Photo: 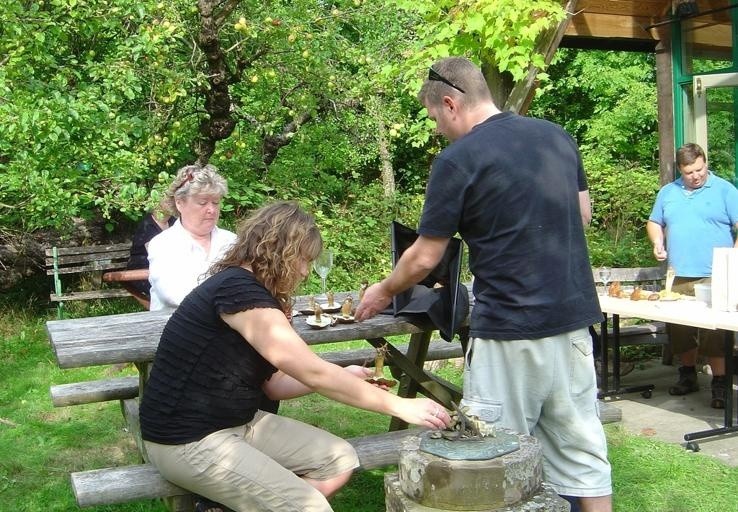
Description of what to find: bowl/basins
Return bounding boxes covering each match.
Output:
[693,283,712,305]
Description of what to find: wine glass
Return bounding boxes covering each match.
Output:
[314,249,332,293]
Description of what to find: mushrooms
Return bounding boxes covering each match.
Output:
[292,280,370,330]
[609,266,681,301]
[365,343,398,387]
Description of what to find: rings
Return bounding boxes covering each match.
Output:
[434,408,440,417]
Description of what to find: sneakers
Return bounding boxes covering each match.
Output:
[712,384,727,408]
[669,377,699,395]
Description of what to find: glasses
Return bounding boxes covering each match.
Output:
[428,66,465,93]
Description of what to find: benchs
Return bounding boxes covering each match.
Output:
[51,340,462,408]
[43,242,142,319]
[69,428,428,508]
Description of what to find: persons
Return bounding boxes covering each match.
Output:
[352,57,613,511]
[140,197,453,511]
[125,190,177,300]
[646,142,738,410]
[145,163,241,309]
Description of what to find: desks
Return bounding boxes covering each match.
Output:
[595,287,738,455]
[45,280,479,512]
